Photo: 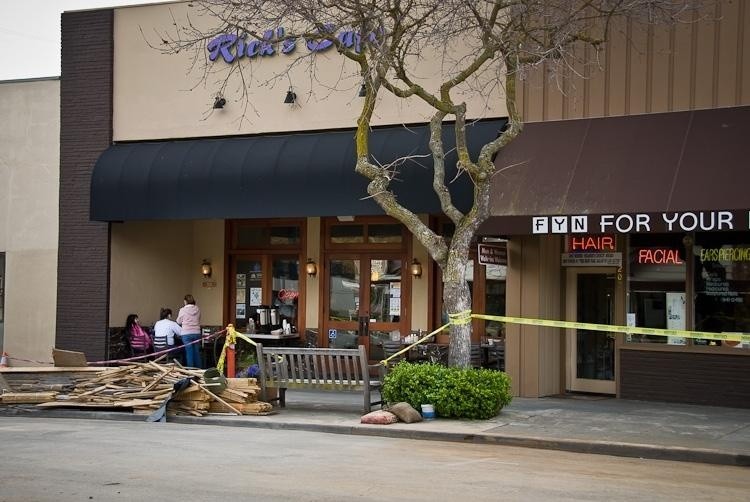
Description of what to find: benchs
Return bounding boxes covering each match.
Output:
[382,342,504,371]
[255,343,388,416]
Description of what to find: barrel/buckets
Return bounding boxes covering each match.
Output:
[421,404,435,420]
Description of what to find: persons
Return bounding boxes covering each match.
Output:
[154,308,181,354]
[125,314,152,350]
[176,294,202,368]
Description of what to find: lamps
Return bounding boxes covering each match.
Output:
[201,259,212,278]
[306,257,316,277]
[358,83,367,97]
[214,96,225,108]
[411,257,422,279]
[283,91,296,102]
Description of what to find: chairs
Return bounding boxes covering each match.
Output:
[127,328,220,368]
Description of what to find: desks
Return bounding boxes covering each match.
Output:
[239,332,299,346]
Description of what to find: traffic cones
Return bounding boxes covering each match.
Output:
[0,352,9,369]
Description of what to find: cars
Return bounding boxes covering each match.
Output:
[330,315,383,358]
